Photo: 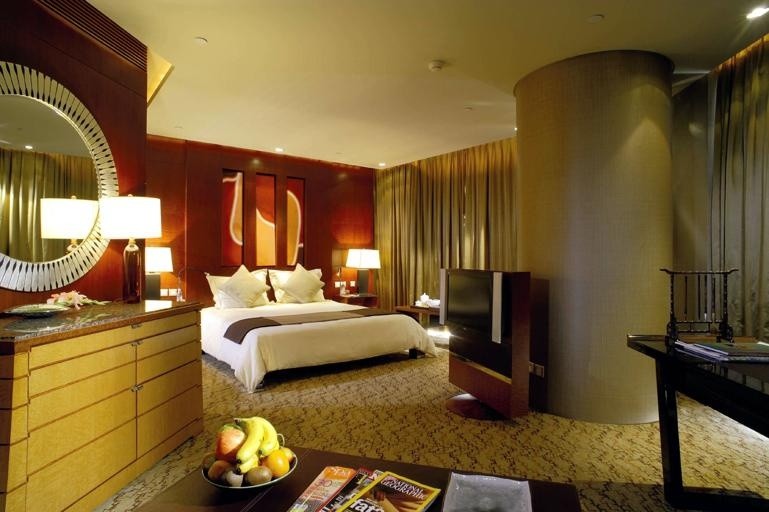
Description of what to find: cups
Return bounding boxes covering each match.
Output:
[415,290,440,307]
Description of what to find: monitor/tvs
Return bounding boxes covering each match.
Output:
[440,268,506,345]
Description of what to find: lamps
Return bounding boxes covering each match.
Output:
[346,249,381,296]
[145,247,173,300]
[102,194,163,303]
[40,196,98,255]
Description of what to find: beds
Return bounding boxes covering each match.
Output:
[198,299,438,393]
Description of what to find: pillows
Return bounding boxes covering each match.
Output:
[204,263,326,309]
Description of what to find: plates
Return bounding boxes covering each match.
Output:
[200,444,297,489]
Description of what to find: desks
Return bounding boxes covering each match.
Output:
[394,306,440,326]
[135,446,582,512]
[627,333,769,512]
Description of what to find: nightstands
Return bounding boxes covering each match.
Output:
[332,295,381,308]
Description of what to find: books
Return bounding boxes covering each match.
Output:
[674,338,769,363]
[283,465,442,512]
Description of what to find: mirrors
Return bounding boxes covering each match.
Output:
[1,62,119,292]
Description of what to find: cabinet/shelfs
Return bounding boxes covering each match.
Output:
[445,271,550,421]
[0,301,216,511]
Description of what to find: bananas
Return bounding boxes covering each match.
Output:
[233,415,279,475]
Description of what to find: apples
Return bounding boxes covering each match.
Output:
[215,425,249,463]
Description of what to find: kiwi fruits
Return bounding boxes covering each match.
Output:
[242,466,273,485]
[220,467,244,487]
